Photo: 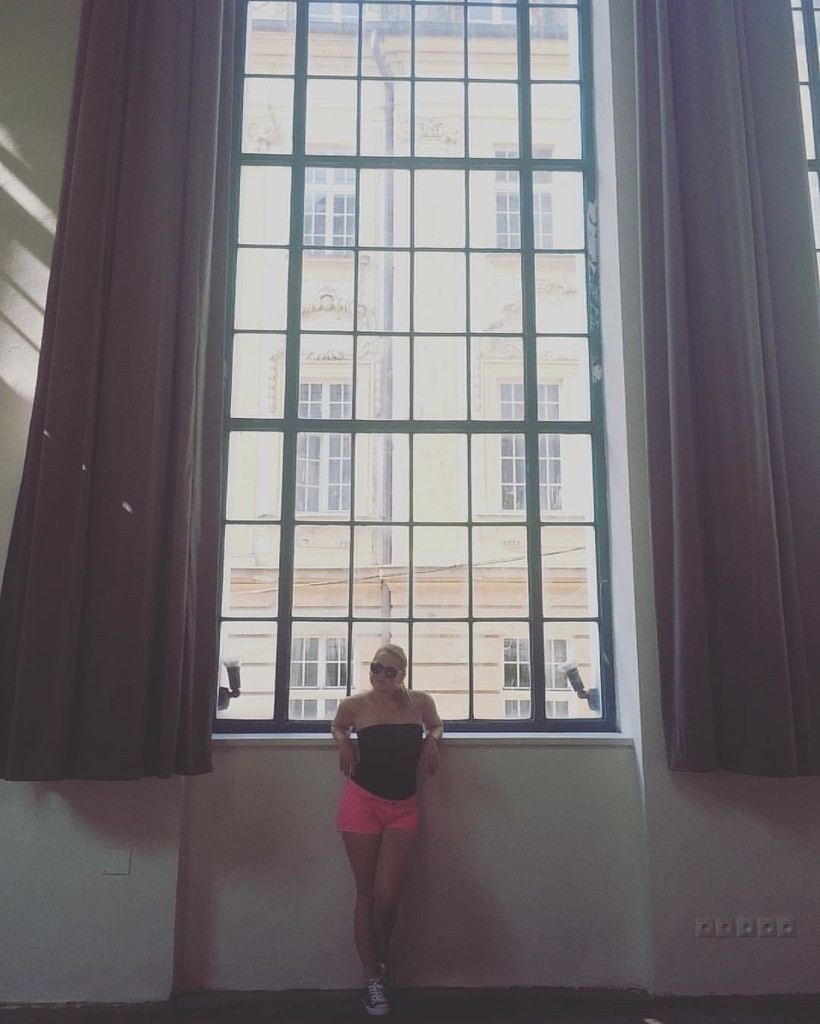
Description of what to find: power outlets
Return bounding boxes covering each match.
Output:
[755,917,777,937]
[715,918,735,938]
[776,916,797,937]
[735,917,756,937]
[695,918,714,936]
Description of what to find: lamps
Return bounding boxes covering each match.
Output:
[217,658,243,711]
[559,659,600,710]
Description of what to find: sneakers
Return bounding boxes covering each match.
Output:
[362,983,391,1016]
[378,962,390,983]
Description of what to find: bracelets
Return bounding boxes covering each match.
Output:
[425,733,439,743]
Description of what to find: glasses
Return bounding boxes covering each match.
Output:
[371,662,402,678]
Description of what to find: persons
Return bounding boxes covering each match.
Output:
[331,644,444,1015]
